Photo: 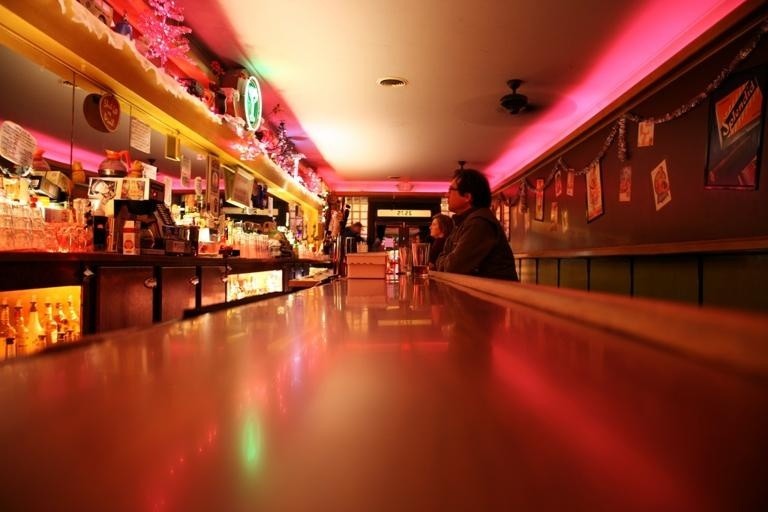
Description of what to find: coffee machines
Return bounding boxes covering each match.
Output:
[113,199,166,255]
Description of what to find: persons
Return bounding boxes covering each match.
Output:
[425,215,454,272]
[345,221,364,244]
[412,233,427,243]
[434,170,520,282]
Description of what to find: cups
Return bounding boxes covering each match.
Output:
[409,276,432,313]
[411,242,431,276]
[0,197,109,250]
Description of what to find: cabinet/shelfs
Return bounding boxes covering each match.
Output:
[0,0,336,361]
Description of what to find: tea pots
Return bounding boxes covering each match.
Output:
[99,151,131,173]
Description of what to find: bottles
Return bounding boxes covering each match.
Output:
[341,255,348,276]
[0,299,82,361]
[177,194,270,255]
